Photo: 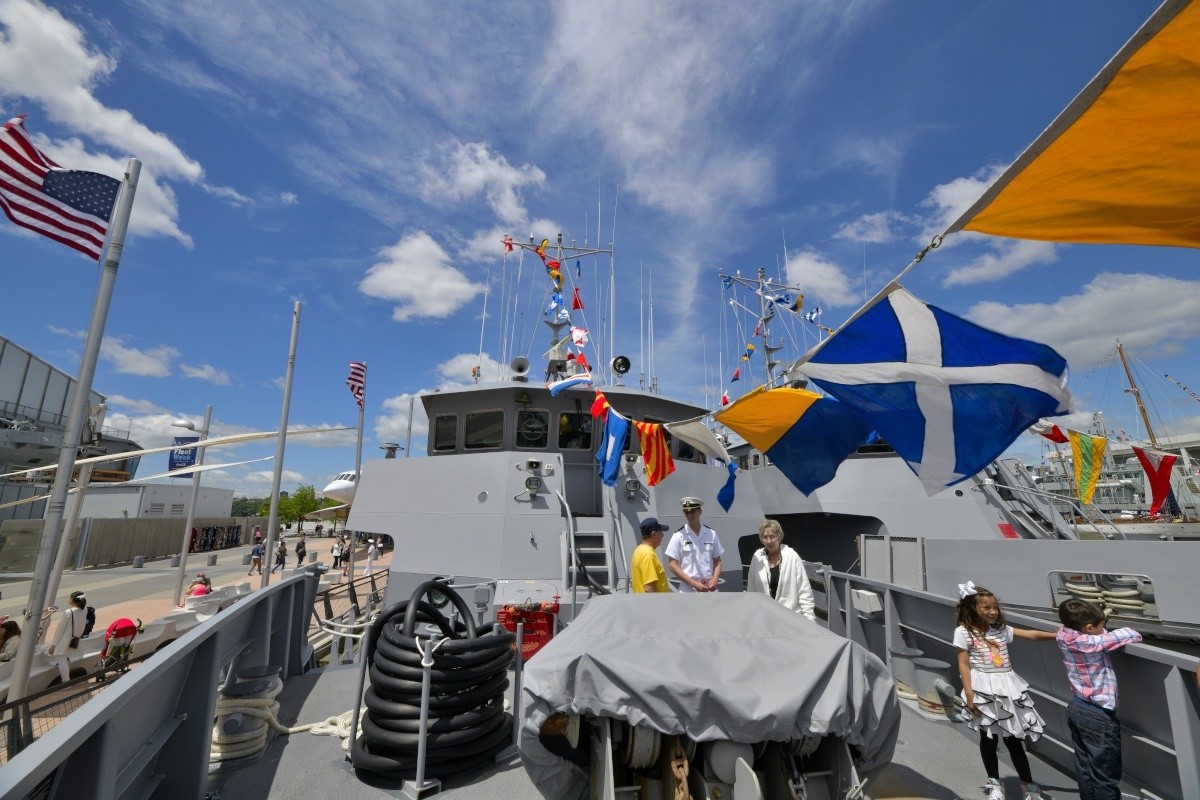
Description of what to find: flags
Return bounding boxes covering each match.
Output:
[1028,420,1069,443]
[710,381,877,497]
[345,361,367,409]
[718,273,834,406]
[1066,429,1108,505]
[961,0,1200,246]
[662,420,741,513]
[0,113,122,260]
[500,238,629,487]
[793,279,1075,497]
[631,420,676,486]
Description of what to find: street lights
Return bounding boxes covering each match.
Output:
[170,404,214,607]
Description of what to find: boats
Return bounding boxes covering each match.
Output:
[1027,337,1200,542]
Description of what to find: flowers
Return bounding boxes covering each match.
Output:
[958,580,976,599]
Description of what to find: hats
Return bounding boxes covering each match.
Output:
[679,497,704,512]
[192,577,203,582]
[368,538,375,544]
[639,518,669,532]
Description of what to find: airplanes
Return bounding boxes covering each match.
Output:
[303,469,362,520]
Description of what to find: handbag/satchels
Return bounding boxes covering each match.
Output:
[69,636,80,648]
[298,548,304,555]
[378,543,383,548]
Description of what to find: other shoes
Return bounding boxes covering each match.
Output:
[258,573,261,574]
[176,605,184,609]
[982,777,1006,800]
[1020,784,1043,800]
[248,573,253,576]
[60,682,71,691]
[55,657,70,665]
[342,574,347,577]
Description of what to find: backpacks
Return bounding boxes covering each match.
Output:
[330,546,334,553]
[80,606,95,638]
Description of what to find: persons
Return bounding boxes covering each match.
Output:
[0,591,9,623]
[295,536,306,568]
[375,535,384,557]
[665,500,724,593]
[953,585,1057,800]
[254,529,263,544]
[247,539,267,576]
[271,542,288,574]
[332,537,351,576]
[747,519,817,623]
[340,530,361,548]
[299,528,305,540]
[314,525,334,538]
[48,591,88,692]
[274,527,287,564]
[176,572,212,608]
[1056,598,1142,800]
[359,540,375,576]
[630,518,668,594]
